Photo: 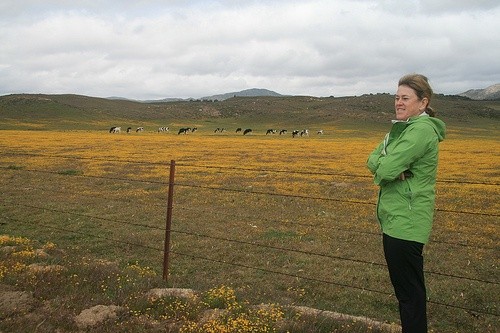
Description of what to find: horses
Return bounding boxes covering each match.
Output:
[178,128,198,135]
[214,127,225,134]
[109,126,144,134]
[157,126,169,134]
[235,127,252,135]
[265,128,324,138]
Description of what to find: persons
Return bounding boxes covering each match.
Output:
[365,74,446,332]
